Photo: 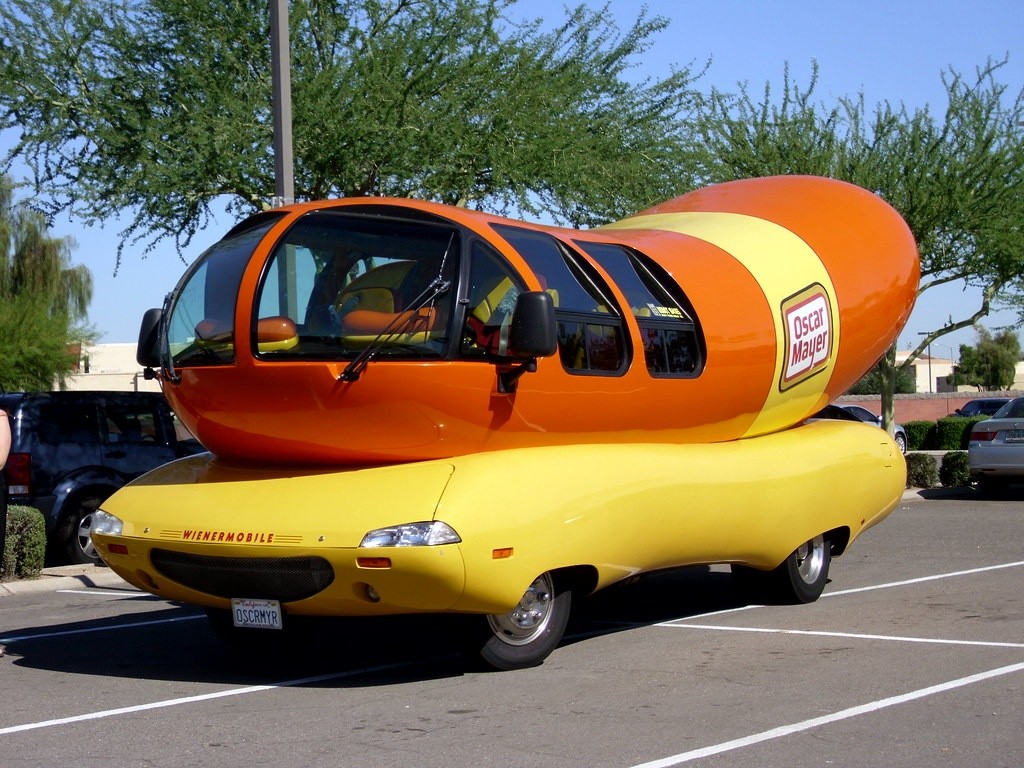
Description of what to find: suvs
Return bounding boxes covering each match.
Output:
[1,390,210,565]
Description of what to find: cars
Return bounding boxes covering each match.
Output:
[955,398,1012,418]
[968,396,1024,493]
[833,403,908,456]
